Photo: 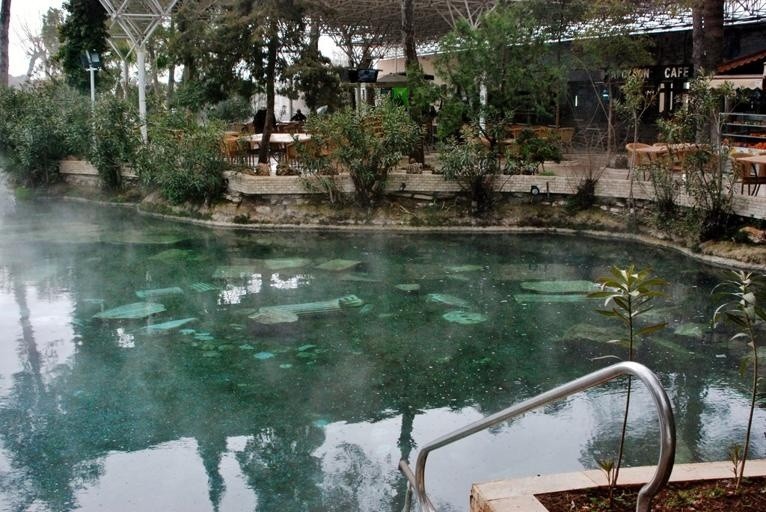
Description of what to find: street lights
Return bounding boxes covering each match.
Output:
[84,48,102,158]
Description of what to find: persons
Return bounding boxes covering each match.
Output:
[290,108,307,128]
[276,105,288,123]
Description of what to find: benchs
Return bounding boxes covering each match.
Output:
[296,124,304,132]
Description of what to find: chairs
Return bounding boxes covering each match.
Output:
[217,134,255,167]
[756,151,766,178]
[284,137,339,169]
[501,127,575,154]
[728,153,757,196]
[626,142,714,183]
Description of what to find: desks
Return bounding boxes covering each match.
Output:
[277,121,299,133]
[242,133,314,163]
[736,155,766,196]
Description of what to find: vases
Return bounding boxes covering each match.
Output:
[346,67,383,83]
